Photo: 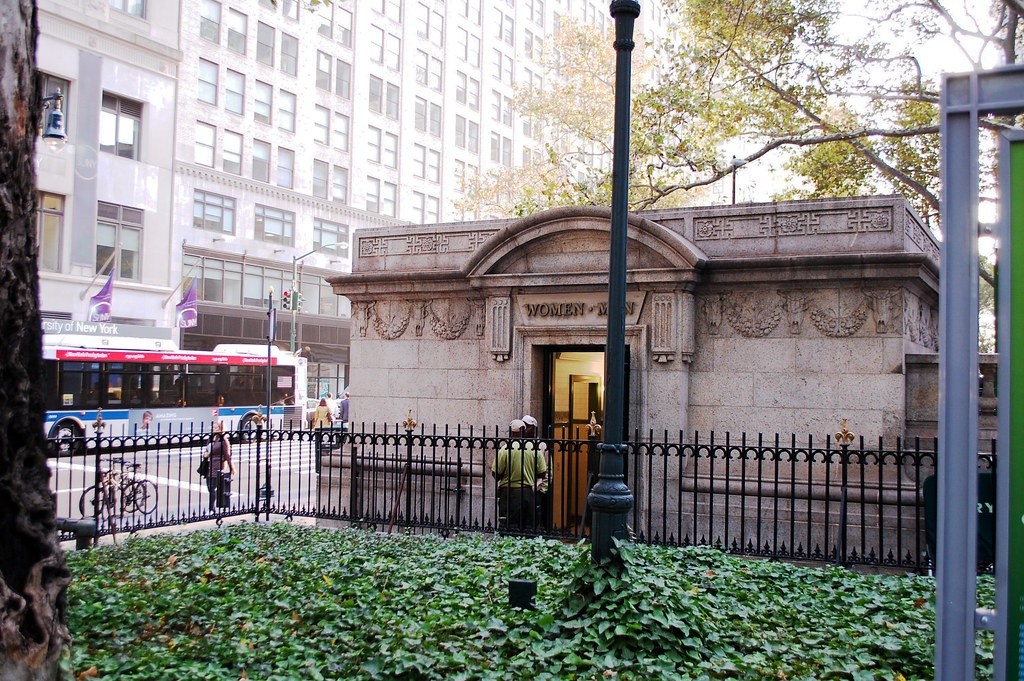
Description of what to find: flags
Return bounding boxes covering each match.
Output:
[173,275,198,329]
[84,269,113,324]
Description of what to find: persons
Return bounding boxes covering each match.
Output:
[311,391,349,432]
[491,415,554,528]
[202,422,237,515]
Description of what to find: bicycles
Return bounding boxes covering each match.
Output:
[78,458,160,519]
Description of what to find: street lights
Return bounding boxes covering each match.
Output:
[289,241,350,353]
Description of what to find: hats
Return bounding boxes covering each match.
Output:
[507,419,526,432]
[522,415,537,427]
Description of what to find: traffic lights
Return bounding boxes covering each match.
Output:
[293,291,302,310]
[283,290,292,310]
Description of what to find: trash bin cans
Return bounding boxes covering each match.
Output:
[316,425,347,474]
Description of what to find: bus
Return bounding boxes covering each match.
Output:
[41,333,311,459]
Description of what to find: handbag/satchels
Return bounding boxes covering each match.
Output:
[327,413,332,422]
[197,457,210,476]
